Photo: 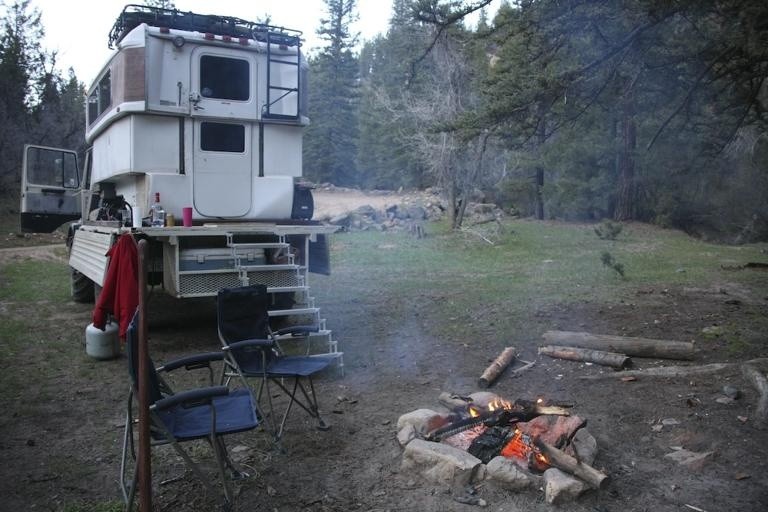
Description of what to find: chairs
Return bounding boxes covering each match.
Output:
[118,305,260,511]
[213,284,333,442]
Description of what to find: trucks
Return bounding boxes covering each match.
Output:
[20,142,345,337]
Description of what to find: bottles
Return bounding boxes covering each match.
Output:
[166,214,175,226]
[151,192,165,228]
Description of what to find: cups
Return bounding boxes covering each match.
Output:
[183,206,192,227]
